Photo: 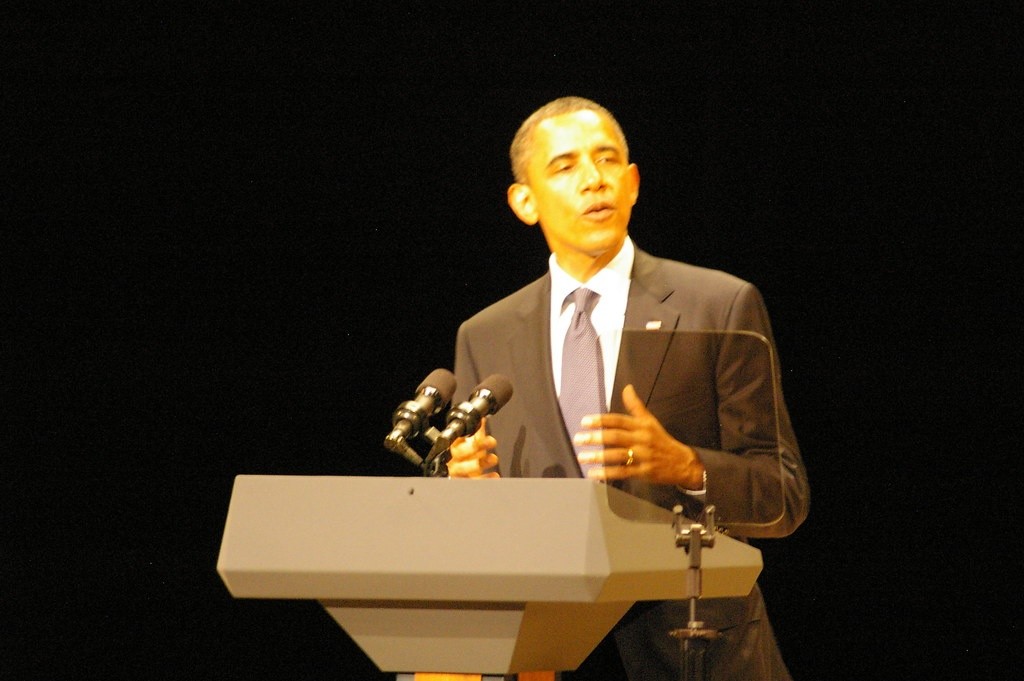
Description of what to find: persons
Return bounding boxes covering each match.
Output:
[445,98,808,680]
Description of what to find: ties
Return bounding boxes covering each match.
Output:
[559,288,609,485]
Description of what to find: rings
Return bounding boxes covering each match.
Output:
[624,445,634,467]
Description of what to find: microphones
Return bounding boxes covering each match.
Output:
[436,374,514,451]
[384,369,457,452]
